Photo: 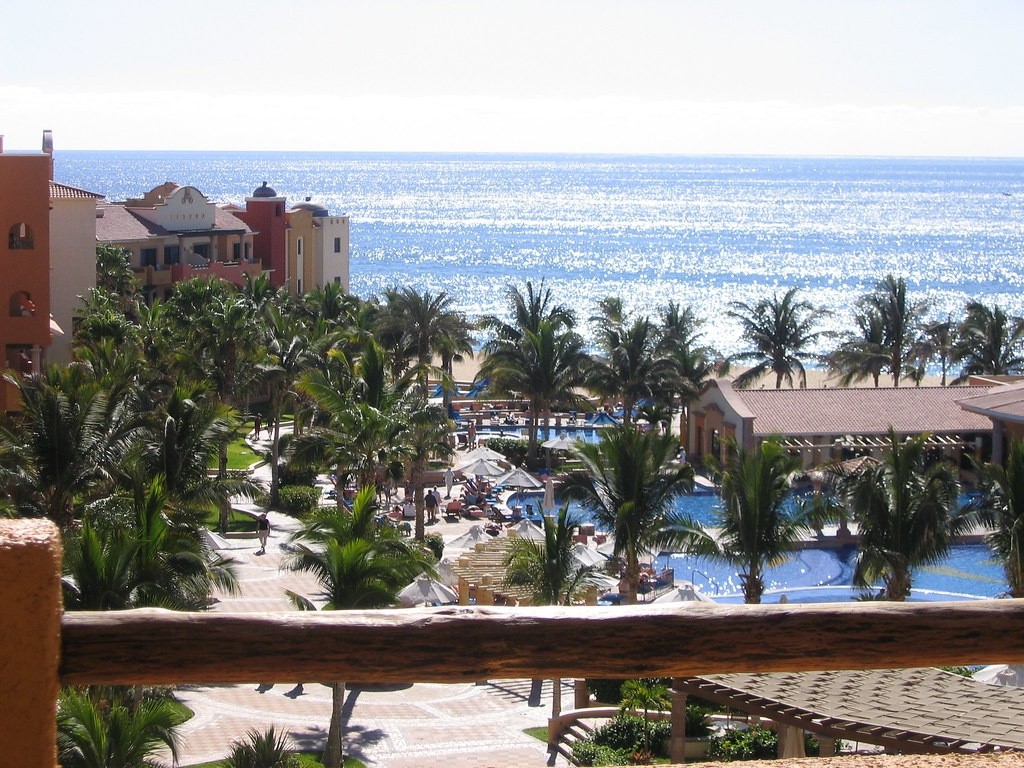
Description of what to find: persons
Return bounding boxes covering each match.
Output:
[386,482,397,503]
[253,416,262,439]
[391,500,415,520]
[466,484,492,504]
[468,423,476,452]
[800,381,804,388]
[443,466,456,498]
[678,446,687,465]
[823,385,827,389]
[267,418,274,440]
[424,486,442,522]
[661,420,670,434]
[256,513,270,553]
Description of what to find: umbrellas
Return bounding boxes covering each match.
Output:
[635,399,654,407]
[452,447,506,475]
[542,431,580,450]
[199,527,230,550]
[613,407,639,418]
[496,468,544,503]
[431,376,489,399]
[589,413,618,425]
[396,518,716,604]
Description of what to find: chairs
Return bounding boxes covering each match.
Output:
[440,478,507,519]
[598,551,657,604]
[329,473,411,535]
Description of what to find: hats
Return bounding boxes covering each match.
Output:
[448,466,451,469]
